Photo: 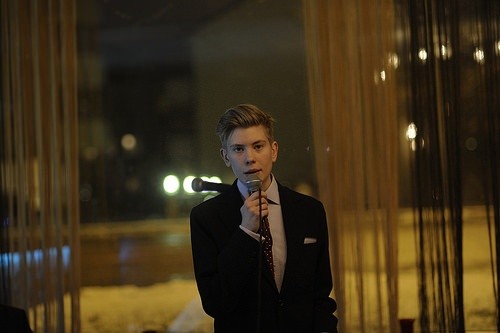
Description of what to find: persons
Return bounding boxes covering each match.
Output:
[190,105,340,333]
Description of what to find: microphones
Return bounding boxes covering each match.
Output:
[192,178,232,193]
[246,175,264,233]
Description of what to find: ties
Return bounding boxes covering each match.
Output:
[248,190,275,283]
